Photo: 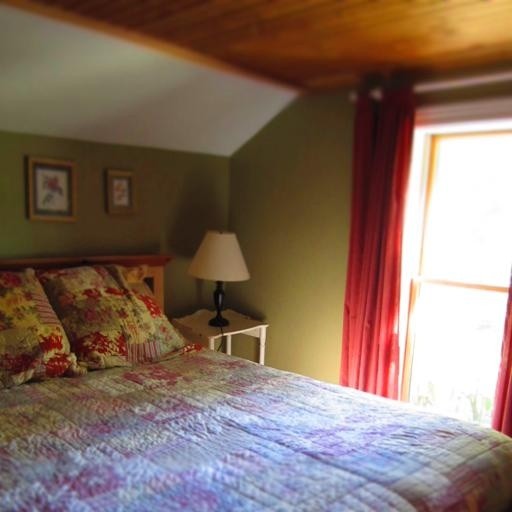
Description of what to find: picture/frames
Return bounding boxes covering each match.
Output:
[23,156,79,224]
[101,164,134,217]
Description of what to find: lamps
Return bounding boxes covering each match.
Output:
[187,228,254,328]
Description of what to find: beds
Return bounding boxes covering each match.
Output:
[0,252,512,512]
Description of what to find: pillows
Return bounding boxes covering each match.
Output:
[34,261,203,373]
[0,265,88,391]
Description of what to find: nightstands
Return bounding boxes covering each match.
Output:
[171,308,270,366]
